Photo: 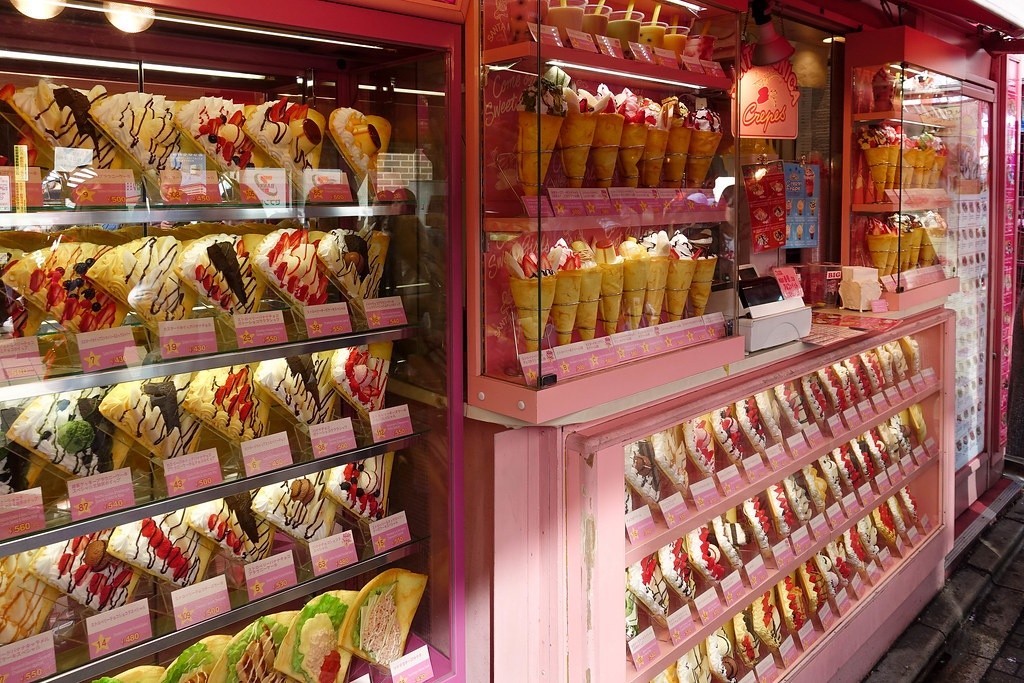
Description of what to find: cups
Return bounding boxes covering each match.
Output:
[605,10,646,61]
[662,26,692,71]
[579,3,614,48]
[640,21,670,60]
[506,0,549,45]
[701,33,718,64]
[685,35,706,65]
[546,0,588,48]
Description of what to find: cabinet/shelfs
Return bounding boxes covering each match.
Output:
[467,0,743,425]
[423,305,955,683]
[839,24,997,520]
[0,0,469,683]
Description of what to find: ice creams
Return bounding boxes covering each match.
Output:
[785,200,816,239]
[504,66,724,356]
[858,67,951,278]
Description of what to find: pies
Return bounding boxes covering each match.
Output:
[88,567,428,683]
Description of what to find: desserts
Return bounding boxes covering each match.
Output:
[548,6,719,61]
[0,78,394,643]
[623,337,928,683]
